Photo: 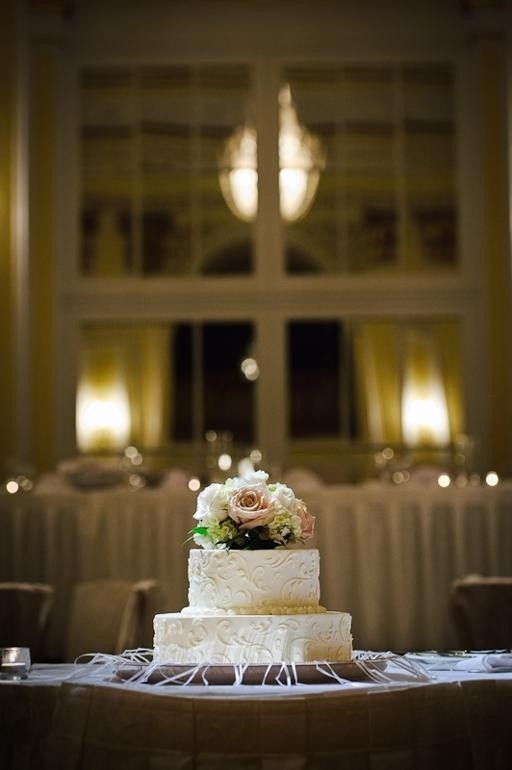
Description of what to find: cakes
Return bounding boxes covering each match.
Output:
[152,548,353,666]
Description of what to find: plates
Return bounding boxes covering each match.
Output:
[112,659,389,683]
[404,650,472,664]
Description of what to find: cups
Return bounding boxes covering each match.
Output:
[1,647,31,681]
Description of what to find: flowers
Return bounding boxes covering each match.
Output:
[180,469,317,555]
